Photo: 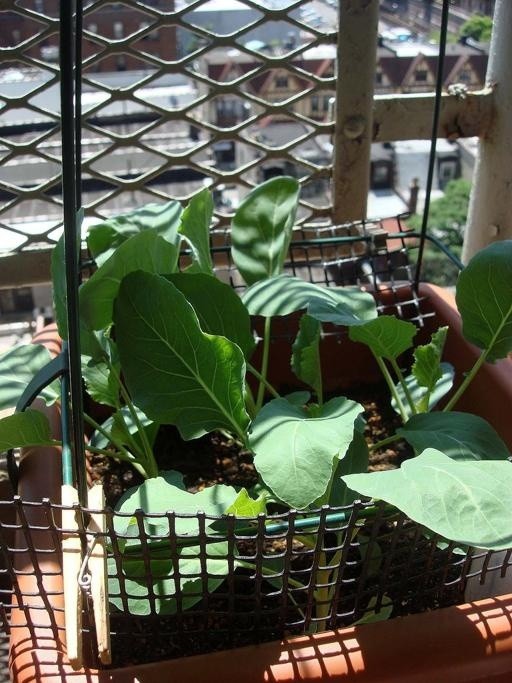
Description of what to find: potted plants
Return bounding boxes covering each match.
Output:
[0,176,512,682]
